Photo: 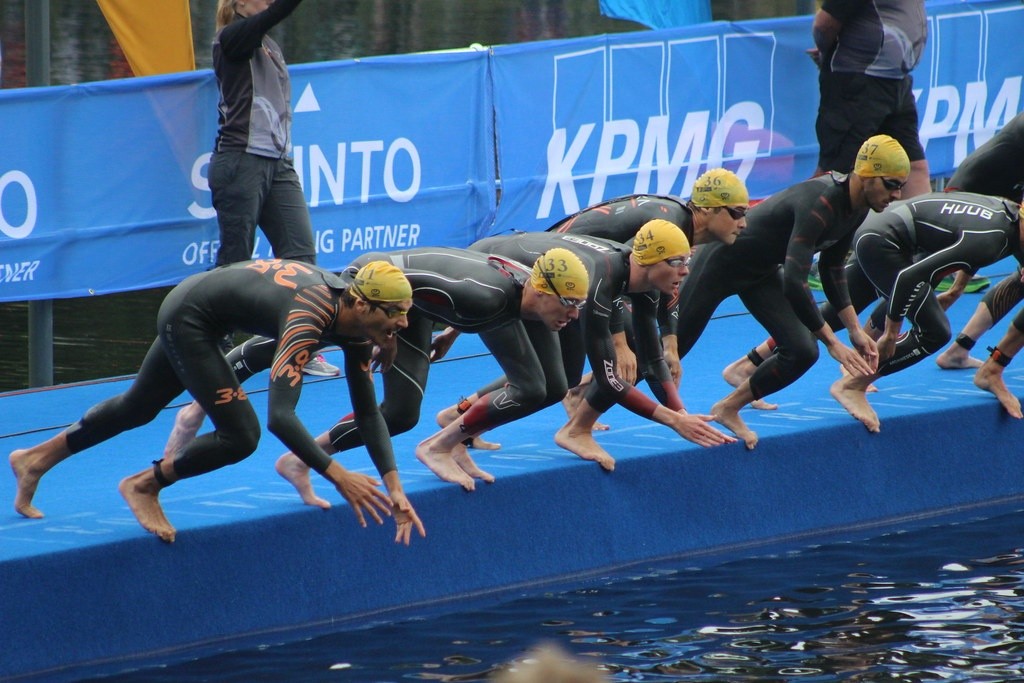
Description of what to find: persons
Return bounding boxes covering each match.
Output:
[208,0,317,267]
[434,112,1023,470]
[160,247,590,509]
[7,260,427,548]
[806,0,931,200]
[415,219,738,491]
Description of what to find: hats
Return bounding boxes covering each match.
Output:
[633,219,690,265]
[349,260,414,303]
[1018,193,1024,219]
[532,248,589,300]
[691,168,750,207]
[853,134,910,177]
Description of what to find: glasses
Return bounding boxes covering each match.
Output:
[370,303,409,318]
[722,206,750,221]
[879,175,907,191]
[559,297,586,310]
[665,256,693,270]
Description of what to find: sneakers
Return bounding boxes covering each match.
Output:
[935,270,991,295]
[302,355,340,377]
[805,259,825,290]
